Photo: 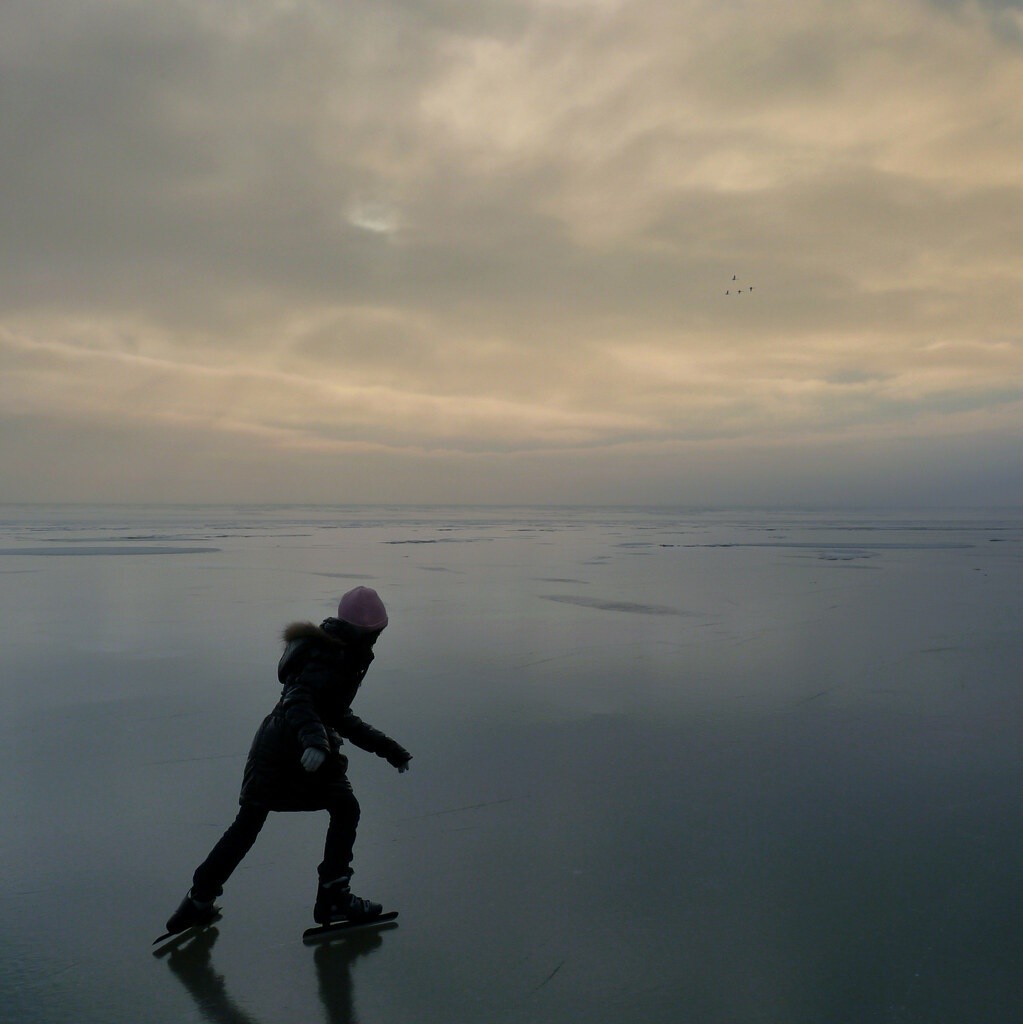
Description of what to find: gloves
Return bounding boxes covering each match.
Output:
[398,761,409,773]
[301,747,326,772]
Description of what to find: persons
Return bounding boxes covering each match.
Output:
[166,587,413,933]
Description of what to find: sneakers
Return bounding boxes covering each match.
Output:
[152,884,223,946]
[303,860,399,938]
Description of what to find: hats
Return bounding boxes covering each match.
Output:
[338,586,388,628]
